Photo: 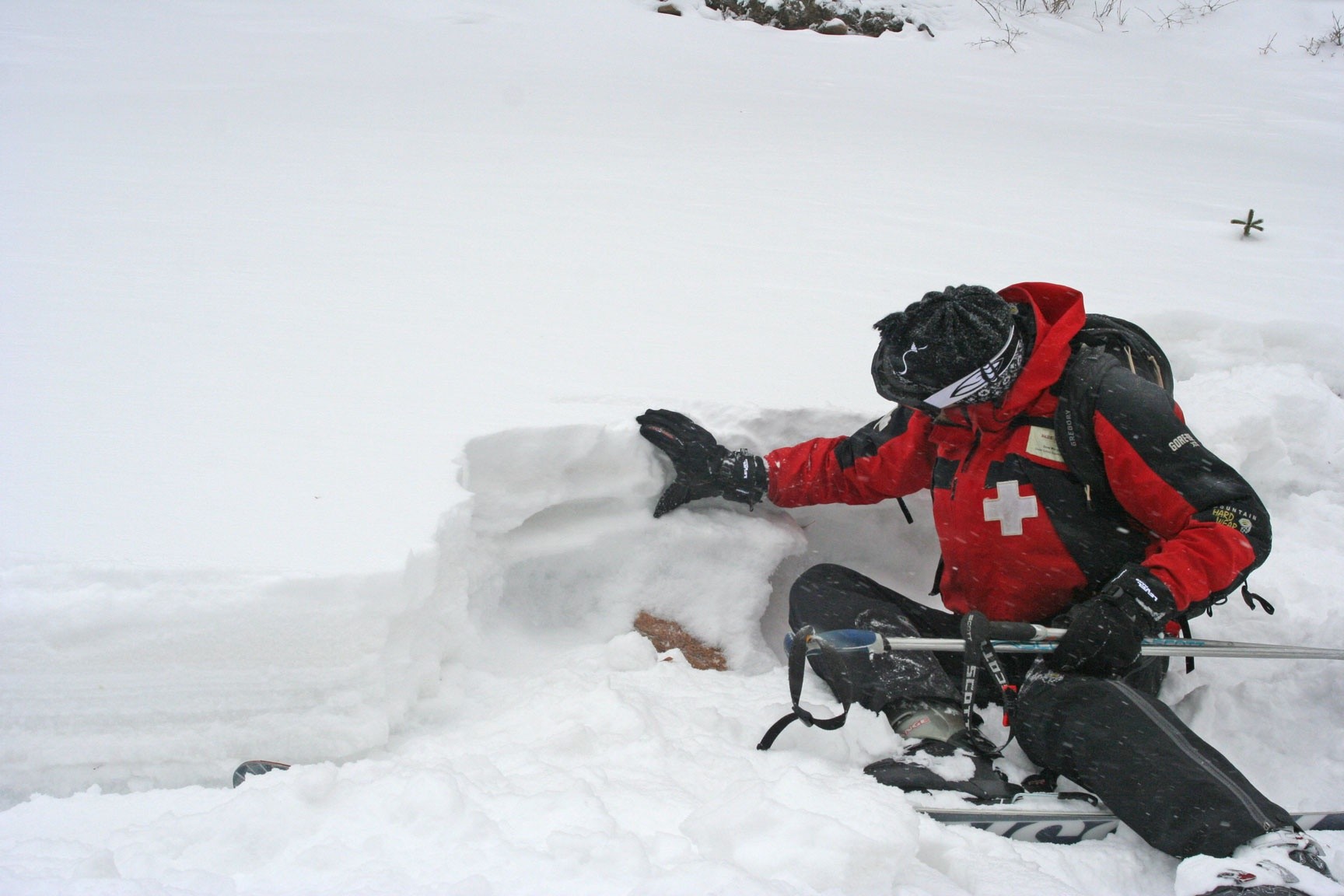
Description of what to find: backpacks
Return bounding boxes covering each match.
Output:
[1054,314,1174,522]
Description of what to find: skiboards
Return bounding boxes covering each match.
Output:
[850,734,1342,894]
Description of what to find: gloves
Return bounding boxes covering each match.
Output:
[635,409,768,519]
[1044,563,1178,681]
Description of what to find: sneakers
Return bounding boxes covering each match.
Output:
[865,704,1023,803]
[1198,826,1339,896]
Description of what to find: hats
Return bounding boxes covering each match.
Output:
[871,285,1023,415]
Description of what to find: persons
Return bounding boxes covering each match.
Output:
[633,282,1344,896]
